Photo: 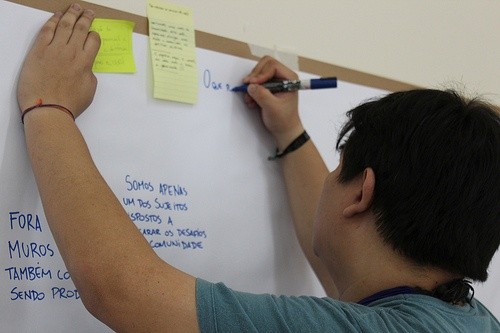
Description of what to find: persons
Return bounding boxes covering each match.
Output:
[17,4,500,333]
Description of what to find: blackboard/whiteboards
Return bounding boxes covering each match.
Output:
[0,0,500,333]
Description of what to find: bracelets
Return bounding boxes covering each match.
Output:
[268,131,310,159]
[21,98,75,125]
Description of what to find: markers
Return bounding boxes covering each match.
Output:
[232,75,338,94]
